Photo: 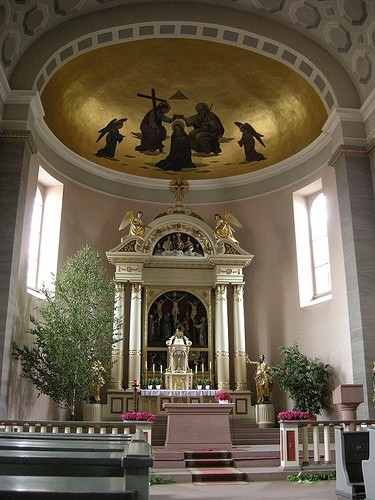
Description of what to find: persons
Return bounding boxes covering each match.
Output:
[211,210,243,238]
[119,211,145,242]
[246,353,277,403]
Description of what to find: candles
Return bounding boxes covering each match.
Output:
[195,365,198,374]
[209,361,212,369]
[160,364,162,373]
[152,364,155,373]
[145,360,147,369]
[202,364,204,372]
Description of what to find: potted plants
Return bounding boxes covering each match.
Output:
[147,380,153,390]
[197,381,203,390]
[266,341,334,427]
[205,381,211,390]
[155,379,161,389]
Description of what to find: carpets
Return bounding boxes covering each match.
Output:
[181,449,251,485]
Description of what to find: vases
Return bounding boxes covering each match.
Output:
[219,400,229,404]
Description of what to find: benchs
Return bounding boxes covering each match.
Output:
[0,432,154,500]
[334,426,370,499]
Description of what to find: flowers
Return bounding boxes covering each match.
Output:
[214,391,233,401]
[279,411,311,420]
[121,411,157,422]
